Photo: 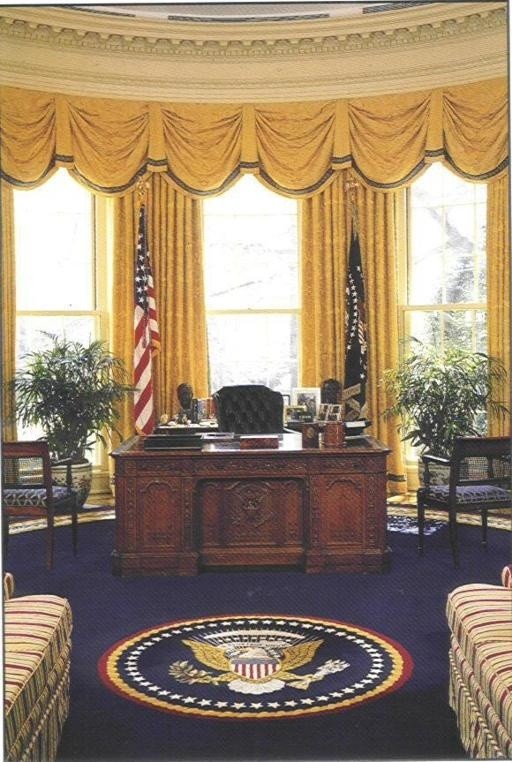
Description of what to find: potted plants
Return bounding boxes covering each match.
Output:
[378,336,510,488]
[1,328,141,510]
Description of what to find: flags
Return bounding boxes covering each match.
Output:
[342,227,375,422]
[131,203,161,436]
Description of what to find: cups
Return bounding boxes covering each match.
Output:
[323,422,344,446]
[302,422,319,448]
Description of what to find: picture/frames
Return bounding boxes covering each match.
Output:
[291,387,322,420]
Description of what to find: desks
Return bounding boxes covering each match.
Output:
[108,434,393,577]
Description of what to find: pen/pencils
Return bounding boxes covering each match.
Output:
[207,434,226,436]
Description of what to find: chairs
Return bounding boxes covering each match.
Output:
[416,435,512,559]
[2,440,79,572]
[211,385,285,434]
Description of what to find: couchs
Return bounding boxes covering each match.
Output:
[443,562,512,760]
[3,573,74,762]
